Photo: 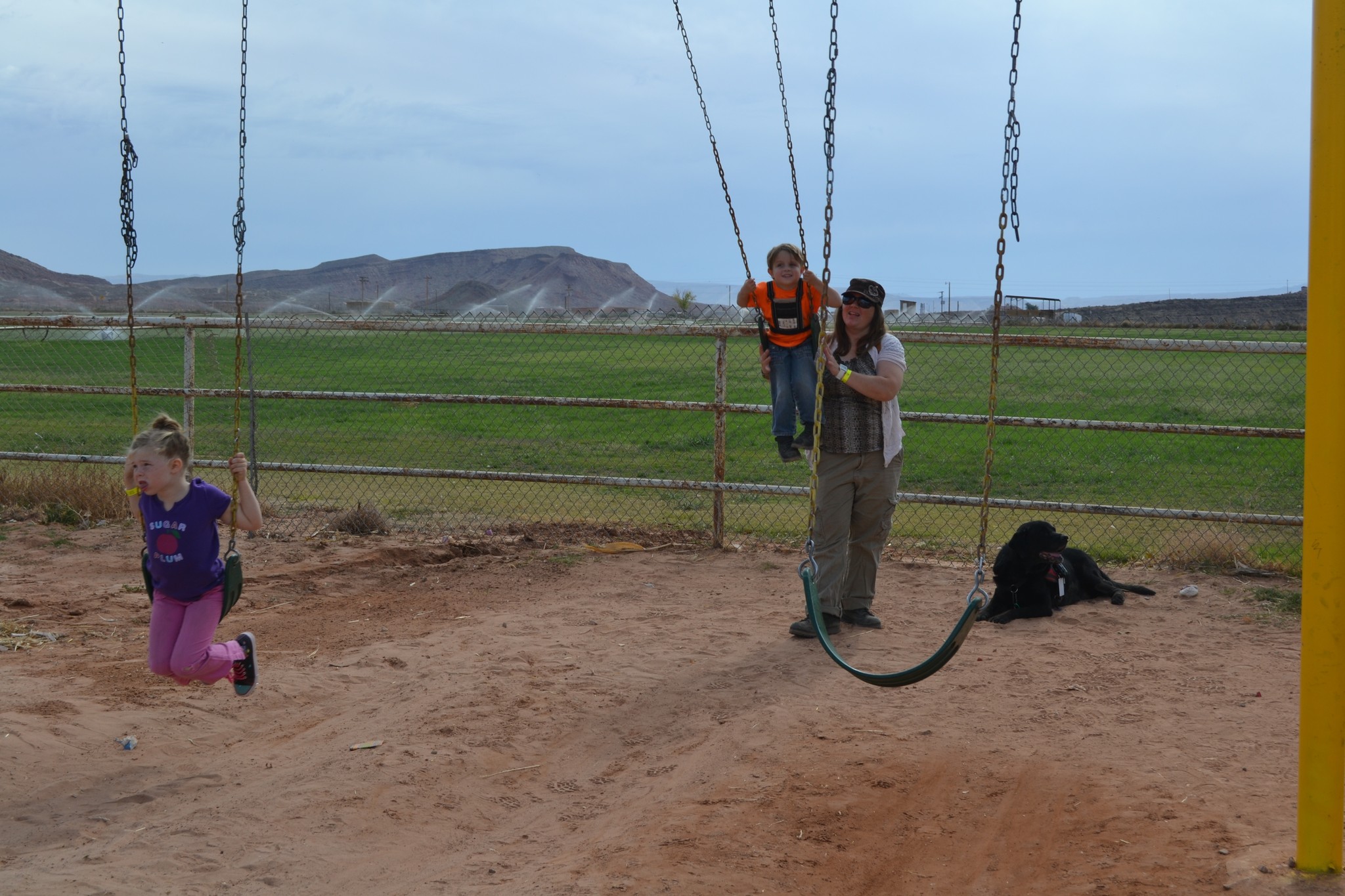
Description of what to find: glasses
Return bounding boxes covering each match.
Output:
[842,293,876,309]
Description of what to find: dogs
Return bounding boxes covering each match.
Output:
[974,520,1155,624]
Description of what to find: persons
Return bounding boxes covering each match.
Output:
[737,243,842,464]
[759,278,909,638]
[122,414,265,698]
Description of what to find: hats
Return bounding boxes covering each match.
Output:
[841,278,885,308]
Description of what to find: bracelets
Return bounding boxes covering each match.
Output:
[123,485,142,497]
[761,369,770,377]
[841,368,852,383]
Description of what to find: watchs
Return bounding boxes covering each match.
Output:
[835,363,847,381]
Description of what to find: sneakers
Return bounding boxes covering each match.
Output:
[841,609,882,628]
[788,611,841,637]
[777,436,802,463]
[790,430,823,450]
[233,631,258,698]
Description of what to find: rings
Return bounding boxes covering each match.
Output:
[829,355,833,360]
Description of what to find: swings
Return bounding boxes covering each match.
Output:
[669,2,835,395]
[112,0,253,643]
[796,0,1027,692]
